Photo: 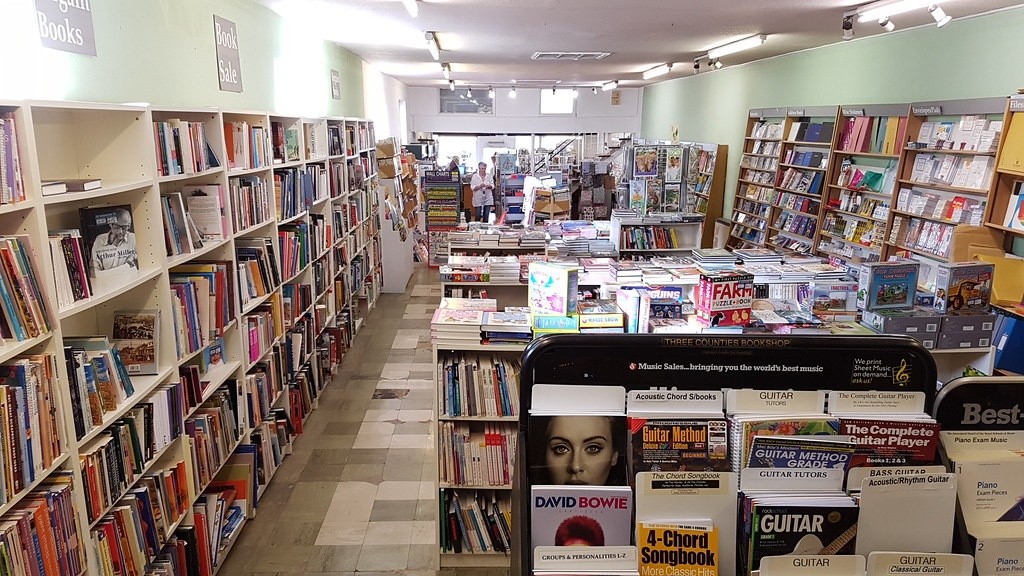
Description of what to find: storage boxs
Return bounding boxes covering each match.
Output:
[932,260,995,317]
[378,156,404,179]
[376,143,401,159]
[858,305,998,349]
[855,260,921,312]
[528,261,578,318]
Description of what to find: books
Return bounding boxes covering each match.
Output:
[0,111,1024,576]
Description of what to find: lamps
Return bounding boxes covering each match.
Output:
[465,85,519,100]
[551,84,599,96]
[441,63,452,81]
[706,31,767,60]
[448,79,455,93]
[642,62,674,81]
[601,79,619,93]
[403,0,420,17]
[693,53,724,75]
[840,0,954,40]
[854,0,952,23]
[424,31,440,61]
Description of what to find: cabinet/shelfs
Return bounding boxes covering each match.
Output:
[725,115,786,254]
[928,348,997,400]
[952,97,1024,320]
[813,104,912,264]
[1,105,96,576]
[695,144,728,250]
[268,108,386,455]
[446,236,618,267]
[767,116,842,259]
[609,217,702,263]
[379,184,415,294]
[150,106,245,573]
[403,138,435,263]
[438,266,613,313]
[30,106,219,576]
[885,97,1009,294]
[495,143,699,217]
[432,344,528,568]
[216,110,293,521]
[423,170,461,226]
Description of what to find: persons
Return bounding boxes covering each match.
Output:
[542,416,619,486]
[89,208,140,278]
[554,515,605,546]
[470,161,494,224]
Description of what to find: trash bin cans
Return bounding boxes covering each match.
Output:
[462,208,471,223]
